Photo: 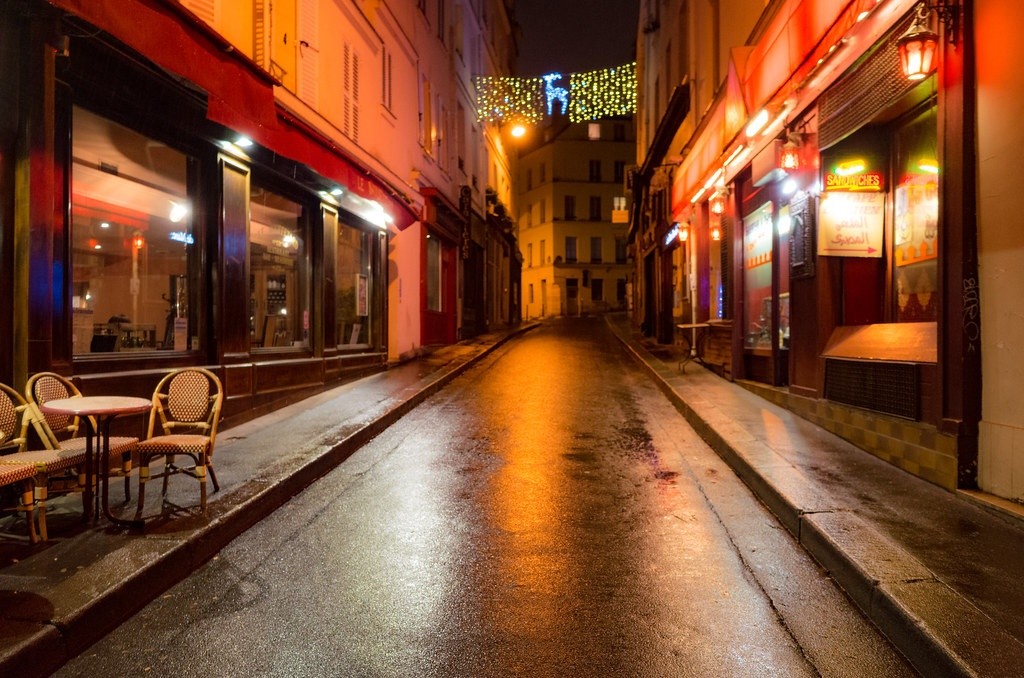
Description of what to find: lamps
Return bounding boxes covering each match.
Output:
[132,230,146,249]
[317,182,343,195]
[896,0,959,82]
[779,114,806,171]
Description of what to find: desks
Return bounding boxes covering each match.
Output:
[676,323,713,373]
[40,395,154,534]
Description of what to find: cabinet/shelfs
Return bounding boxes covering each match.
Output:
[92,322,157,351]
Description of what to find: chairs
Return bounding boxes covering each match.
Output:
[133,367,224,522]
[0,464,37,546]
[25,372,140,518]
[0,382,89,544]
